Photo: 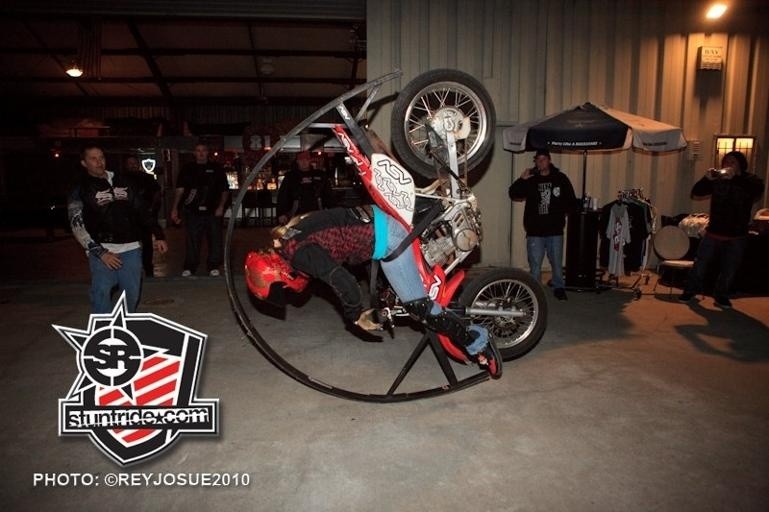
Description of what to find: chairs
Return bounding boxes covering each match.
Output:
[652,225,705,302]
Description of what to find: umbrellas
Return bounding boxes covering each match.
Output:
[500,101,689,214]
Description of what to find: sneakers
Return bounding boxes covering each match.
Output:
[210,269,220,277]
[476,324,503,379]
[181,270,191,277]
[715,295,731,308]
[554,285,568,302]
[678,291,697,302]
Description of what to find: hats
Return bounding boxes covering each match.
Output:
[534,149,551,159]
[728,151,747,171]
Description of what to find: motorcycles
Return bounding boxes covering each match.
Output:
[220,65,551,407]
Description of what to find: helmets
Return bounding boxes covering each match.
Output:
[243,247,311,300]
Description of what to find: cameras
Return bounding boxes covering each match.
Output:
[711,170,721,177]
[533,170,540,176]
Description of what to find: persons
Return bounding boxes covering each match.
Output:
[509,151,579,301]
[69,145,168,321]
[169,143,231,277]
[276,151,334,226]
[679,149,765,309]
[242,204,504,378]
[117,155,163,281]
